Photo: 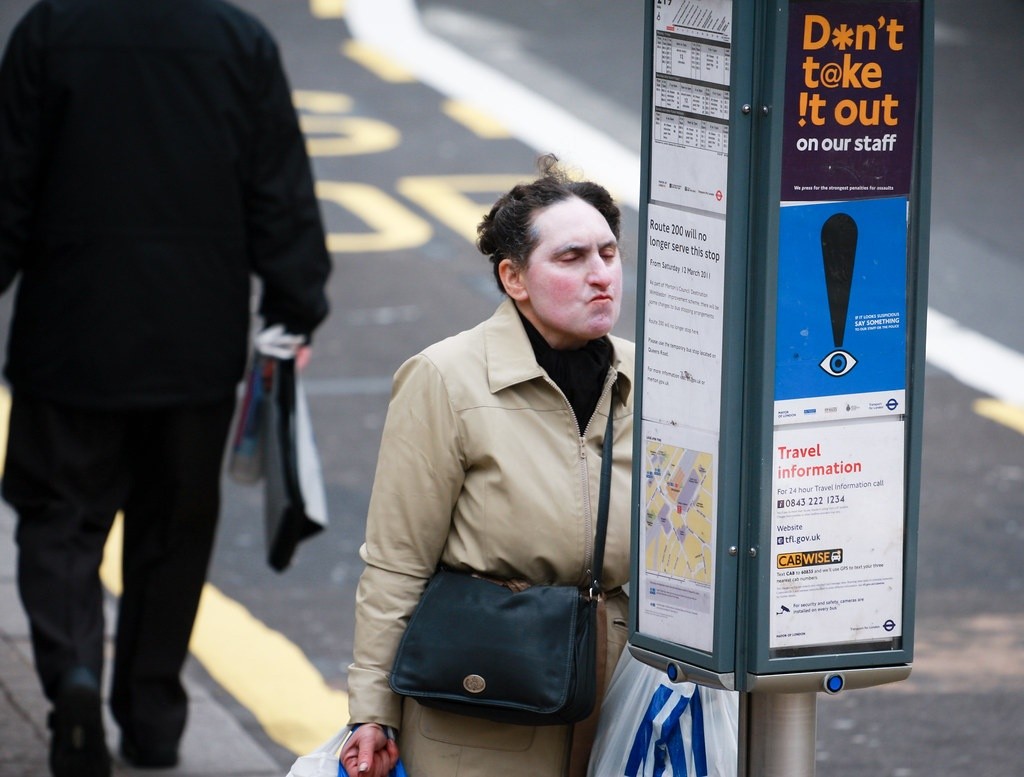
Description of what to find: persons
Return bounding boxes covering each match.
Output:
[0,0,333,777]
[338,155,636,777]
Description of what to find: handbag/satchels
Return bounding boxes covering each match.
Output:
[387,574,614,728]
